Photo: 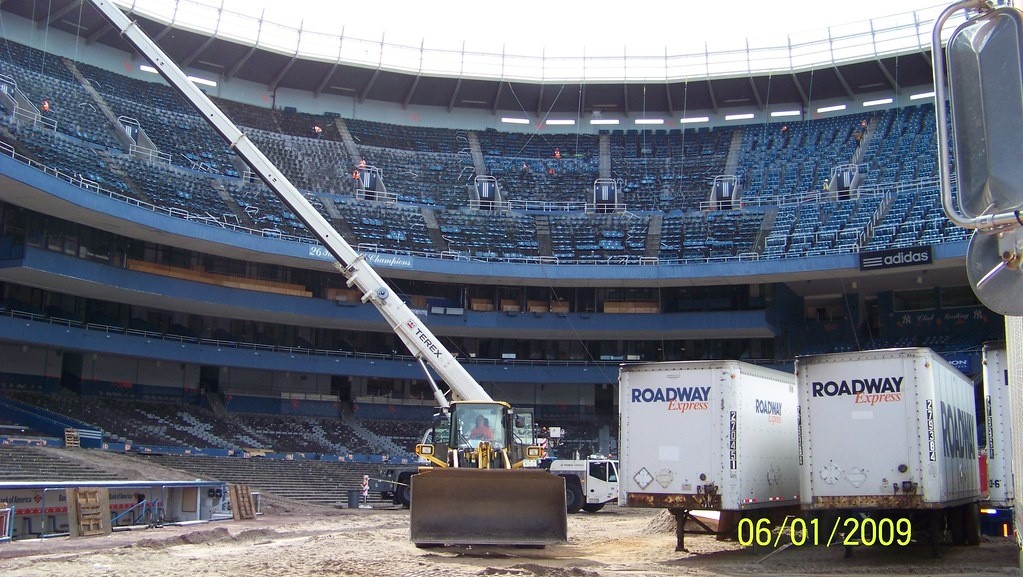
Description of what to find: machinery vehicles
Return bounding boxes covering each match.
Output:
[85,1,618,549]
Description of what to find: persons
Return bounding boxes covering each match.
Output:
[359,155,366,169]
[780,126,789,138]
[823,179,829,191]
[42,98,49,116]
[555,147,560,158]
[855,117,868,148]
[470,415,493,441]
[352,168,359,188]
[312,126,322,140]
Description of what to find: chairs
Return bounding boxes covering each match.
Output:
[0,382,621,461]
[0,36,973,263]
